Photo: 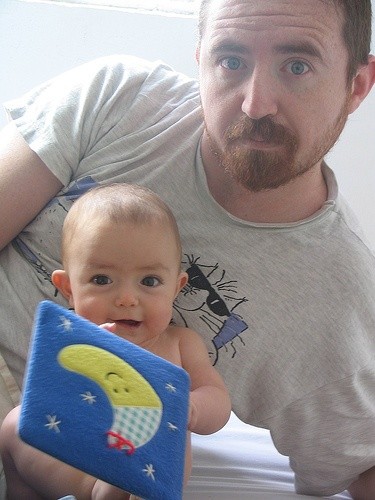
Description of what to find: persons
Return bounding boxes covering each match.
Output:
[0,2,374,500]
[0,182,230,500]
